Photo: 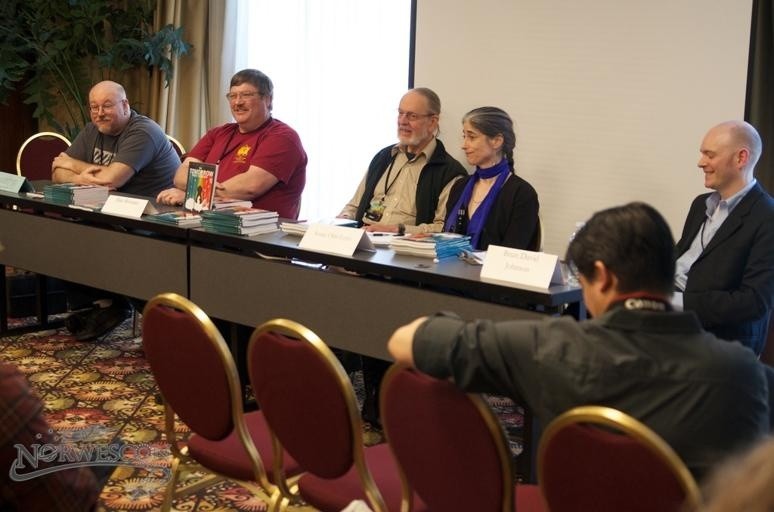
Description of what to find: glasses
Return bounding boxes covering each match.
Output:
[89,100,121,110]
[407,113,434,120]
[227,91,257,99]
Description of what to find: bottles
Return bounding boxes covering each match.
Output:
[568,220,586,289]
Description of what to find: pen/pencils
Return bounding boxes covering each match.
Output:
[373,233,406,236]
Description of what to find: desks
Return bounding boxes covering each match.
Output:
[187,213,589,486]
[0,181,216,336]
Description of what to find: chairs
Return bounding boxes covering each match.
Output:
[245,316,408,512]
[538,407,705,510]
[379,359,547,510]
[13,132,72,179]
[124,135,185,335]
[140,291,310,512]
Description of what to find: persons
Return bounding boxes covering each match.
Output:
[333,86,470,447]
[442,105,539,251]
[389,200,774,488]
[661,119,774,351]
[155,68,311,207]
[50,79,183,341]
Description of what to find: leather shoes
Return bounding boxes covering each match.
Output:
[66,303,131,340]
